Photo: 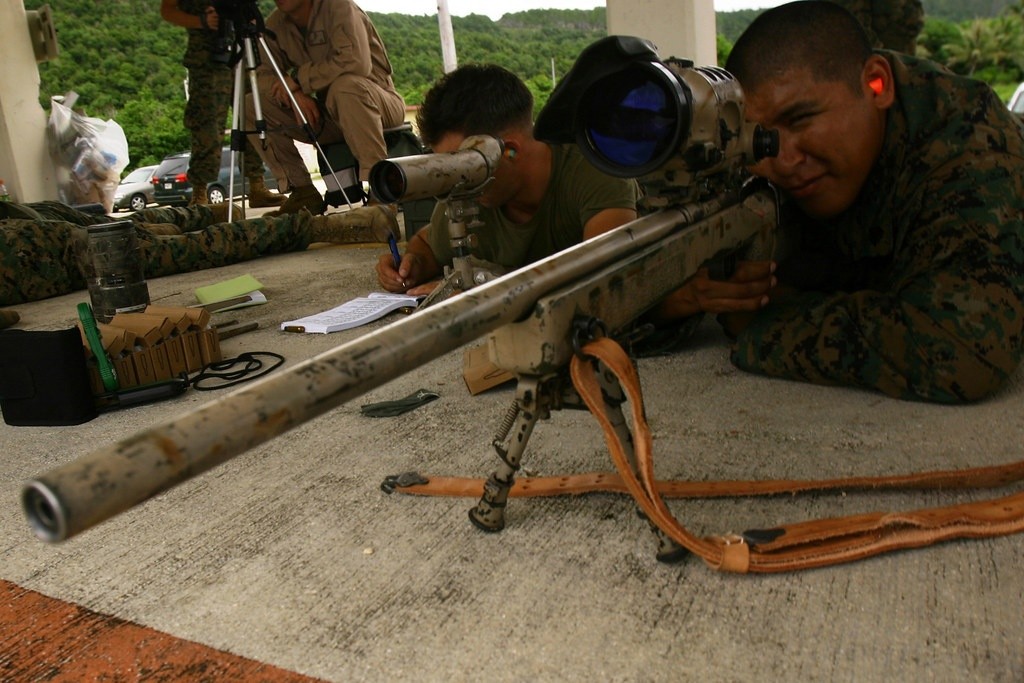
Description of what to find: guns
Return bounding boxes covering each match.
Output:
[21,34,786,561]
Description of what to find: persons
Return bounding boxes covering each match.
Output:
[616,0,1024,403]
[377,64,644,293]
[0,199,401,305]
[256,1,405,215]
[161,0,288,206]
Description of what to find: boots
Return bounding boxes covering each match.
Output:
[309,206,401,245]
[249,175,287,208]
[187,183,208,209]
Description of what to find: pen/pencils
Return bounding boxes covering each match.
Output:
[385,230,407,288]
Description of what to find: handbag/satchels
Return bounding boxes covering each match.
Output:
[0,326,99,427]
[46,96,129,215]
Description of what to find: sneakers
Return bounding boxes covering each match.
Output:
[204,202,244,222]
[136,222,180,237]
[262,184,326,219]
[363,189,399,218]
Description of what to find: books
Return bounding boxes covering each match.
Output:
[185,273,266,314]
[281,288,426,334]
